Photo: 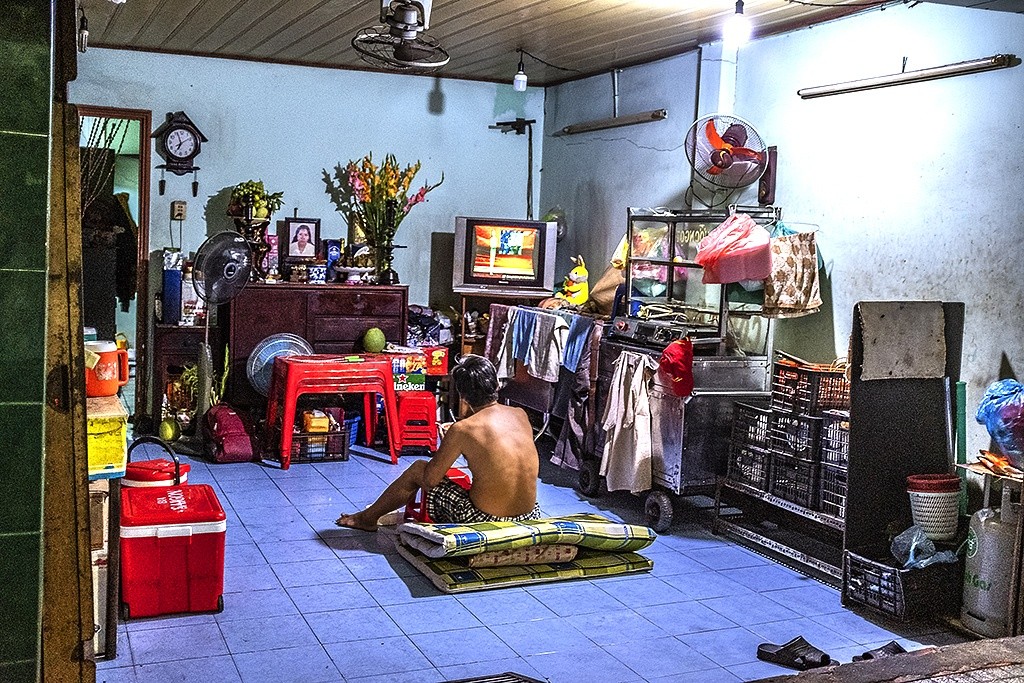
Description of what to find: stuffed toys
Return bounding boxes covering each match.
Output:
[555,254,589,305]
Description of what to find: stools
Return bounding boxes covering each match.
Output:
[395,391,472,522]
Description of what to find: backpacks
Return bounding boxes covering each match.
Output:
[207,402,261,462]
[658,337,695,398]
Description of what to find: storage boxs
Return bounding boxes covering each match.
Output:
[161,247,206,325]
[384,346,449,391]
[272,410,361,465]
[120,484,227,621]
[261,228,341,282]
[727,363,965,618]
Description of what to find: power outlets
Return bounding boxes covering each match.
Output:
[173,202,186,219]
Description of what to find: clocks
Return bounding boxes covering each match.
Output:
[149,111,208,197]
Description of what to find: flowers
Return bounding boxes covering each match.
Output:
[343,151,445,272]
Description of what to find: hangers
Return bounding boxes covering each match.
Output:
[762,205,820,233]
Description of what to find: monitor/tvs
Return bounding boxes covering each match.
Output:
[452,217,558,300]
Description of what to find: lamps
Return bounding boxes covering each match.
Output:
[796,53,1010,99]
[513,50,668,135]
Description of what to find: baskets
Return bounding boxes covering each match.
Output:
[340,415,362,447]
[290,431,351,465]
[907,474,962,542]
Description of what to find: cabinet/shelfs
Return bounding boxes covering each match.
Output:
[625,205,781,356]
[152,279,409,433]
[458,292,552,419]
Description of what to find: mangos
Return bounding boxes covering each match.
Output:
[253,195,268,218]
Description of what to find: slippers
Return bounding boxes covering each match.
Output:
[852,641,906,662]
[757,636,840,671]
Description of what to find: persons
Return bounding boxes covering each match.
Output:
[336,355,539,531]
[290,225,315,256]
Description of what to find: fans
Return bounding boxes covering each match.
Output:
[685,113,777,205]
[171,230,253,455]
[246,333,314,400]
[352,0,450,75]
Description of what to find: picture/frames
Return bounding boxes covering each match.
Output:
[284,217,321,262]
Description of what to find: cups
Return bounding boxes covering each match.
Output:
[84,340,129,397]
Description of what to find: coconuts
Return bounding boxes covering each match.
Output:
[158,419,181,441]
[362,327,386,353]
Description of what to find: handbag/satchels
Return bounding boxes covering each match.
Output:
[975,378,1024,471]
[693,213,773,284]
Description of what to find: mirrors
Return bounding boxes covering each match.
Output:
[74,104,153,419]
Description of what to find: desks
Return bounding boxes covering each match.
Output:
[267,353,401,470]
[86,394,128,660]
[483,304,611,446]
